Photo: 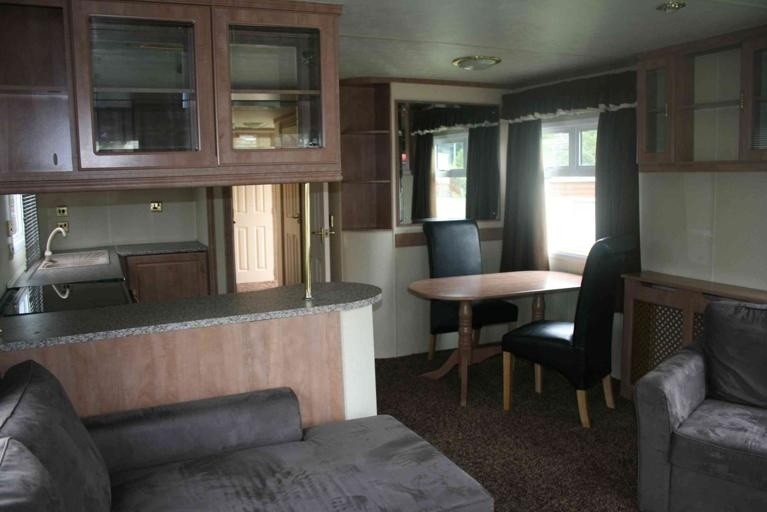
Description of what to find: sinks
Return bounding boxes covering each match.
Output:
[40,248,110,270]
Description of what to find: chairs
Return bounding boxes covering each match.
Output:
[501,231,639,429]
[422,219,518,381]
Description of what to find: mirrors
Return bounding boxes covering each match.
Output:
[395,98,500,226]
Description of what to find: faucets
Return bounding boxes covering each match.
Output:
[45,226,67,257]
[44,272,72,300]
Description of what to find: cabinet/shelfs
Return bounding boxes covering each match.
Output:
[638,21,766,171]
[68,1,344,187]
[117,242,207,302]
[341,77,391,230]
[0,0,77,194]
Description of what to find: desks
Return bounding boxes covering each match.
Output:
[408,268,585,406]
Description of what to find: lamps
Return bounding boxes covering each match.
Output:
[454,54,501,72]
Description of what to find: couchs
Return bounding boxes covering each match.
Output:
[1,362,496,511]
[635,297,767,510]
